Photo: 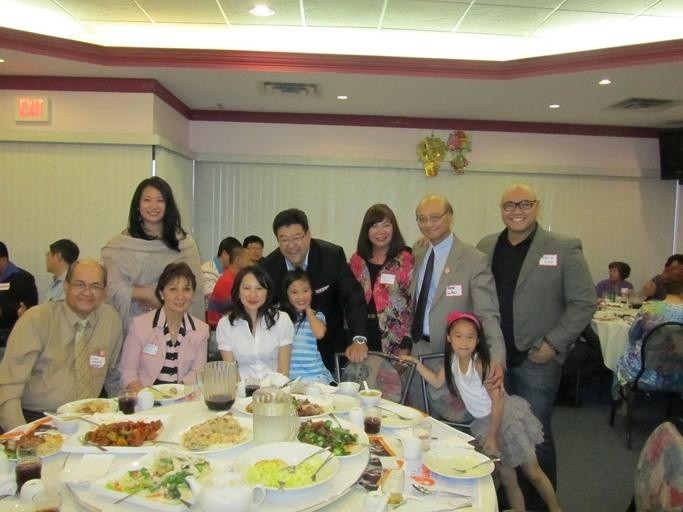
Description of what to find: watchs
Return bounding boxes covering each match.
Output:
[353,337,367,345]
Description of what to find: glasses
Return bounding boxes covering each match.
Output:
[501,200,535,213]
[417,211,446,226]
[278,234,305,247]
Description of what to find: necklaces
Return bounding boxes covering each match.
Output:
[168,314,181,335]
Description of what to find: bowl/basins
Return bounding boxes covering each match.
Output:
[339,381,361,396]
[19,478,45,506]
[358,390,382,405]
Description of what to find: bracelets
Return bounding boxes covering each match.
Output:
[544,337,560,356]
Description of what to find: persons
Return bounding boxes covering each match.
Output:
[199,235,264,331]
[349,204,414,374]
[596,254,683,417]
[0,239,39,359]
[216,266,295,384]
[258,208,369,383]
[0,260,125,434]
[280,271,335,384]
[16,240,79,317]
[475,184,597,512]
[101,177,206,337]
[405,194,507,420]
[119,262,210,395]
[399,312,562,512]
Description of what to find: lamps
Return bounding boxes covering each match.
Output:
[416,129,473,178]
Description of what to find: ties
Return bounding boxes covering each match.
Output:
[410,248,433,343]
[72,321,94,400]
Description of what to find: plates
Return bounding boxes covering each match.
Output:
[139,383,195,405]
[168,410,256,454]
[1,431,67,461]
[296,415,369,460]
[364,403,426,430]
[57,397,120,418]
[422,447,496,480]
[234,441,340,491]
[61,414,173,455]
[396,415,478,446]
[236,393,332,419]
[90,449,233,512]
[316,393,360,414]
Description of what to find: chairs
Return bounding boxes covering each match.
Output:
[626,418,682,512]
[608,322,683,449]
[418,354,477,430]
[562,342,602,407]
[334,350,417,405]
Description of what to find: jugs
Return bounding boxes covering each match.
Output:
[254,389,298,445]
[184,470,267,512]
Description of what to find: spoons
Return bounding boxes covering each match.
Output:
[411,483,472,499]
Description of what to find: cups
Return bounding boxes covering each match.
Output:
[363,407,382,435]
[117,389,135,415]
[14,441,43,495]
[195,361,238,411]
[32,491,63,511]
[381,467,405,508]
[411,422,433,451]
